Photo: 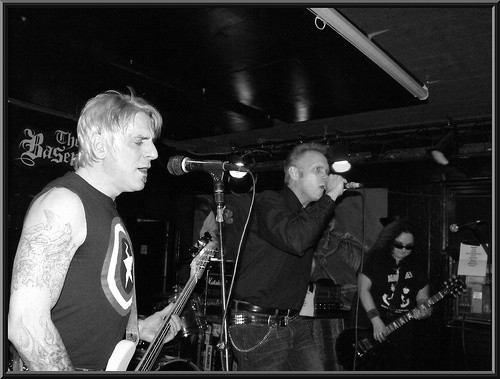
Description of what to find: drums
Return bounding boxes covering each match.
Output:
[166,293,208,341]
[206,258,236,284]
[127,349,202,371]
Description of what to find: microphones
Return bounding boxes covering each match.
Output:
[166,154,249,176]
[449,218,486,232]
[323,181,361,190]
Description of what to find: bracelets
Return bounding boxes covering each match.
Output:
[366,308,380,321]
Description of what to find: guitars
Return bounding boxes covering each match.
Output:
[137,240,218,373]
[336,275,468,371]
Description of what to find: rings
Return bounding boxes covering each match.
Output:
[379,333,382,336]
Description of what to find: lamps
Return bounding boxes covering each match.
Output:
[329,129,353,174]
[429,122,467,167]
[308,6,429,105]
[228,148,248,178]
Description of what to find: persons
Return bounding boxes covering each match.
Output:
[355,224,434,343]
[6,90,182,372]
[226,142,347,372]
